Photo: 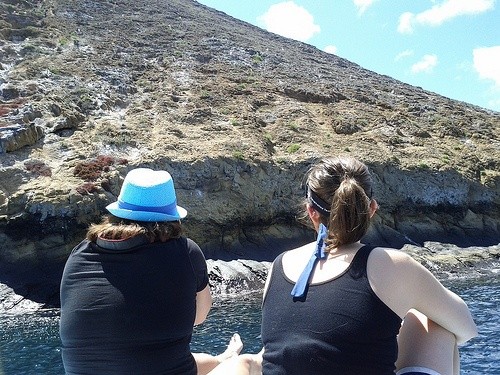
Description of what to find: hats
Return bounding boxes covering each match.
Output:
[105,168,188,222]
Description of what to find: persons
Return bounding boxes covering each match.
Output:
[60,168,264,375]
[261,155,477,375]
